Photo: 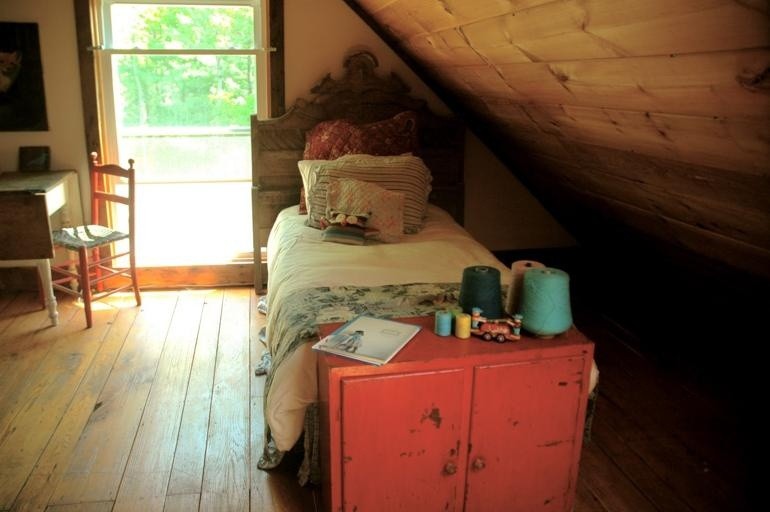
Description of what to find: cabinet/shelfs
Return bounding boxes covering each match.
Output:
[315,314,594,511]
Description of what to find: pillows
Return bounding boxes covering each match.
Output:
[291,109,440,248]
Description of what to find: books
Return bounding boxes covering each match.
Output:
[311,314,422,366]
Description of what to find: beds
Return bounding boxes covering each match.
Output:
[247,42,600,487]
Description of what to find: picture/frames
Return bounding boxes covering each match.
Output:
[17,146,50,171]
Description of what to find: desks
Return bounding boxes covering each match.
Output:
[0,168,86,329]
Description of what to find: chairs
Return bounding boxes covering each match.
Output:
[36,152,142,330]
